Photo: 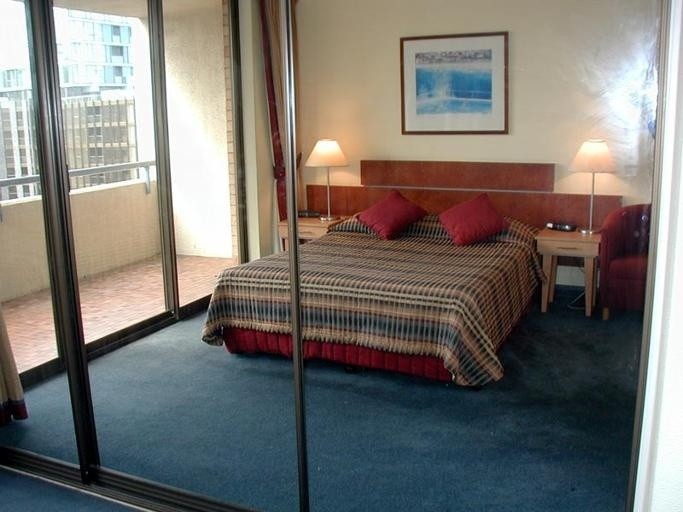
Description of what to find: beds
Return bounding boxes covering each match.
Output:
[201,160,555,386]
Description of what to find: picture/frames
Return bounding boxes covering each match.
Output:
[400,32,507,134]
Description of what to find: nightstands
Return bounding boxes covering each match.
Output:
[534,226,602,316]
[279,216,344,252]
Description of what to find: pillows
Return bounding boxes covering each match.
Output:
[439,192,510,246]
[356,189,429,239]
[617,211,650,256]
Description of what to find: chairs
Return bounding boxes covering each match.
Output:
[599,204,651,321]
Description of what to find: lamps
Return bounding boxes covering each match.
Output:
[305,138,351,221]
[568,139,616,233]
